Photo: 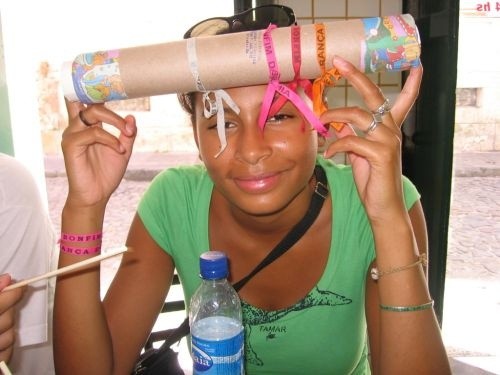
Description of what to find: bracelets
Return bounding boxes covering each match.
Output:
[59,244,102,256]
[61,232,102,243]
[370,255,427,280]
[374,301,437,314]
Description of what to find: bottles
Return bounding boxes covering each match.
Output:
[188,251,245,375]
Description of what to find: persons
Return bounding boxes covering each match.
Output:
[55,5,452,375]
[0,152,55,375]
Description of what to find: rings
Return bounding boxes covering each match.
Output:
[372,98,392,115]
[366,116,382,134]
[78,110,92,128]
